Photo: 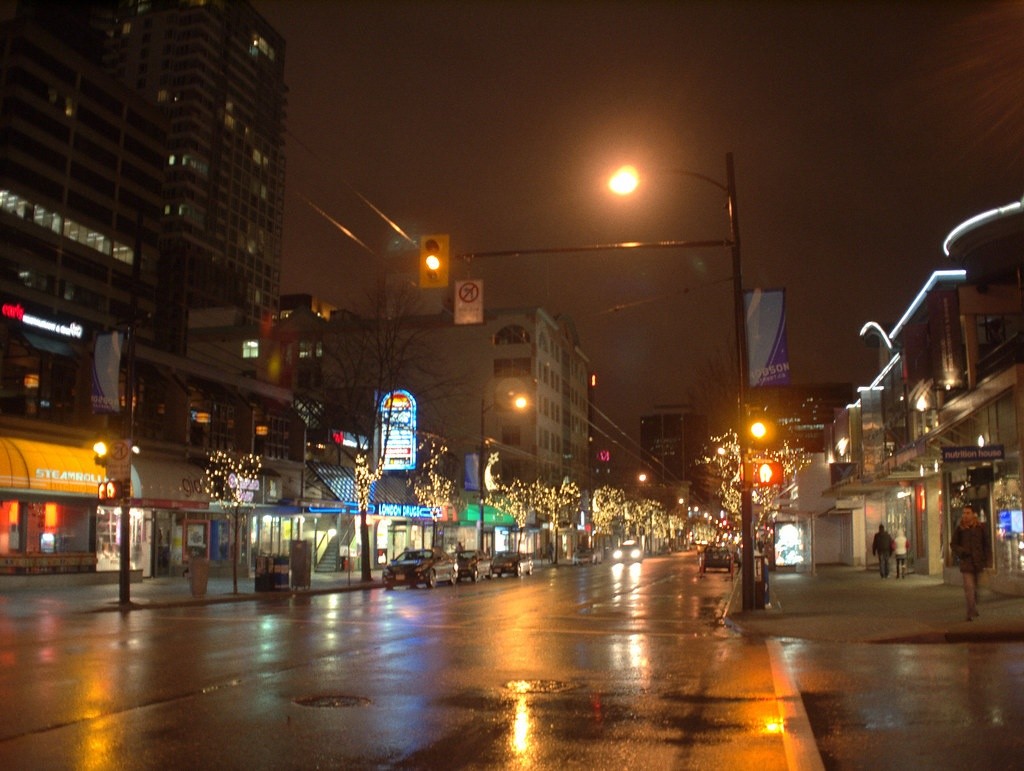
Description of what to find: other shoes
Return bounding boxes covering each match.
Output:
[968,611,979,620]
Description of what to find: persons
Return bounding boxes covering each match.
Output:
[871,524,894,579]
[892,528,910,580]
[455,542,463,552]
[951,506,994,621]
[547,542,555,563]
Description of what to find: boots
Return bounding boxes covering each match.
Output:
[896,569,899,578]
[902,568,905,578]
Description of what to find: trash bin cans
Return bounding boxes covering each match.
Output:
[253,553,290,592]
[752,554,770,609]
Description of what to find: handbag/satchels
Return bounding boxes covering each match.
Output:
[906,539,910,548]
[890,543,896,551]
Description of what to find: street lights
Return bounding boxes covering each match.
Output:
[607,147,775,609]
[478,389,527,553]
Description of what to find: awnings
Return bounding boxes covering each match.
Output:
[0,428,212,509]
[456,504,514,526]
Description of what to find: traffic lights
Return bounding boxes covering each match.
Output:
[97,482,121,502]
[421,233,451,288]
[741,463,783,486]
[93,429,119,467]
[746,408,774,452]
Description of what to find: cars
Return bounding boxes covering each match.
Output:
[572,547,602,565]
[729,543,743,567]
[382,547,459,590]
[612,540,643,563]
[490,553,534,576]
[697,542,734,573]
[448,550,494,582]
